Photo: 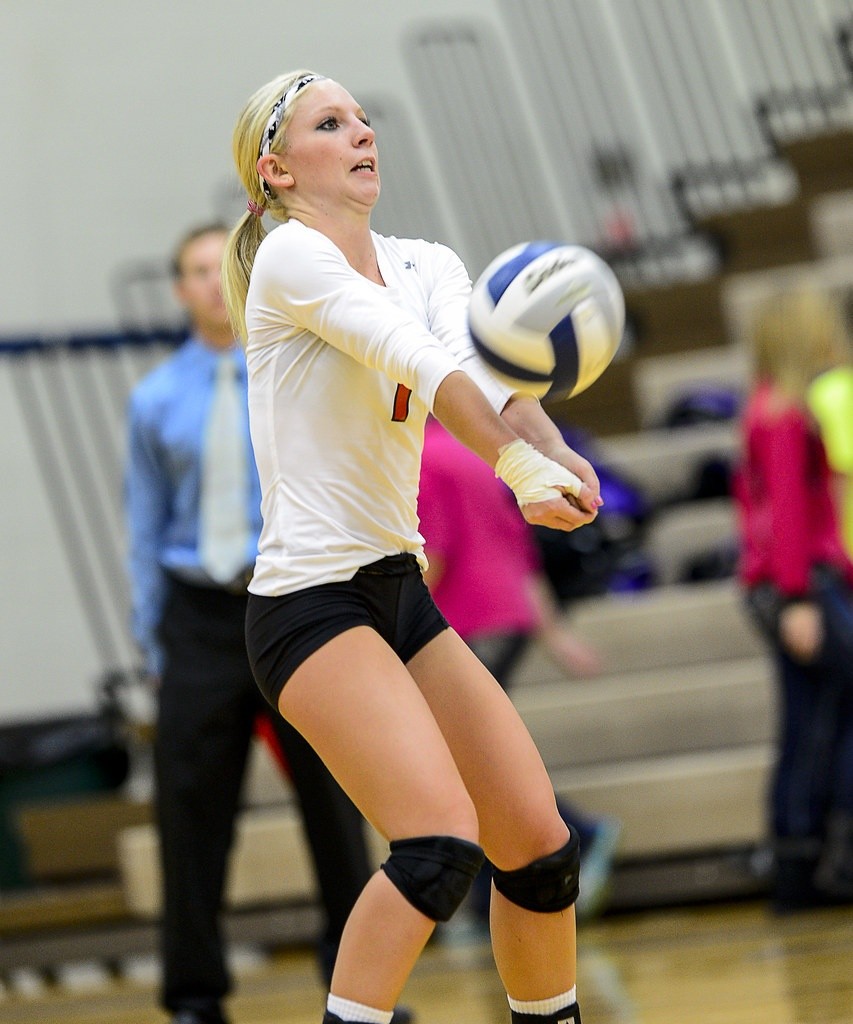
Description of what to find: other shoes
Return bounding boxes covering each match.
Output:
[781,885,853,909]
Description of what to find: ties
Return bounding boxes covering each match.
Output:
[197,356,249,583]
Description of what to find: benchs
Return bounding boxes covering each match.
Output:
[111,185,852,926]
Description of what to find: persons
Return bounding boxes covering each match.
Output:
[727,273,853,911]
[408,409,625,973]
[114,219,409,1023]
[231,71,609,1022]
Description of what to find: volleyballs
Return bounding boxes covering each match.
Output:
[462,235,631,407]
